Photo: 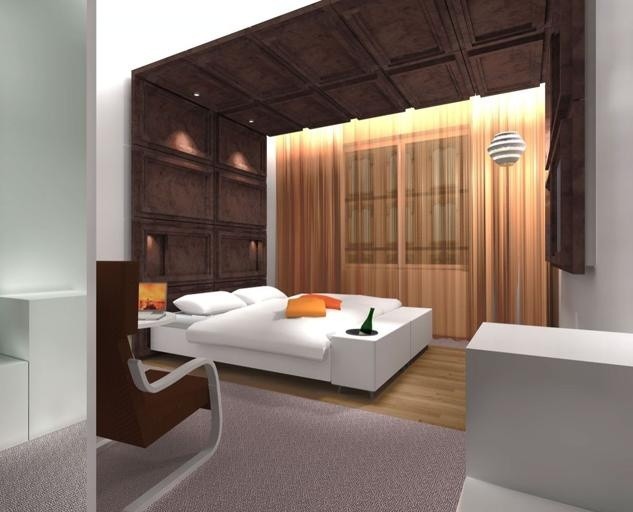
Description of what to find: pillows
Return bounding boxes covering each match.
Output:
[171,286,342,319]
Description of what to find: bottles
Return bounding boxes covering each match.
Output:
[358,305,377,337]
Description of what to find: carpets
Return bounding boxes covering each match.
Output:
[94,378,465,511]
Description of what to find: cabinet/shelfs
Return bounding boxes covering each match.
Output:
[326,307,432,395]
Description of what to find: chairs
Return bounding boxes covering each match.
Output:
[94,260,223,510]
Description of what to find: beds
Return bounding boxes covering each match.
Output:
[150,292,400,392]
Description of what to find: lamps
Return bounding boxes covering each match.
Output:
[487,132,524,325]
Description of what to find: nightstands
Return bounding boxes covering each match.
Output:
[126,312,172,358]
